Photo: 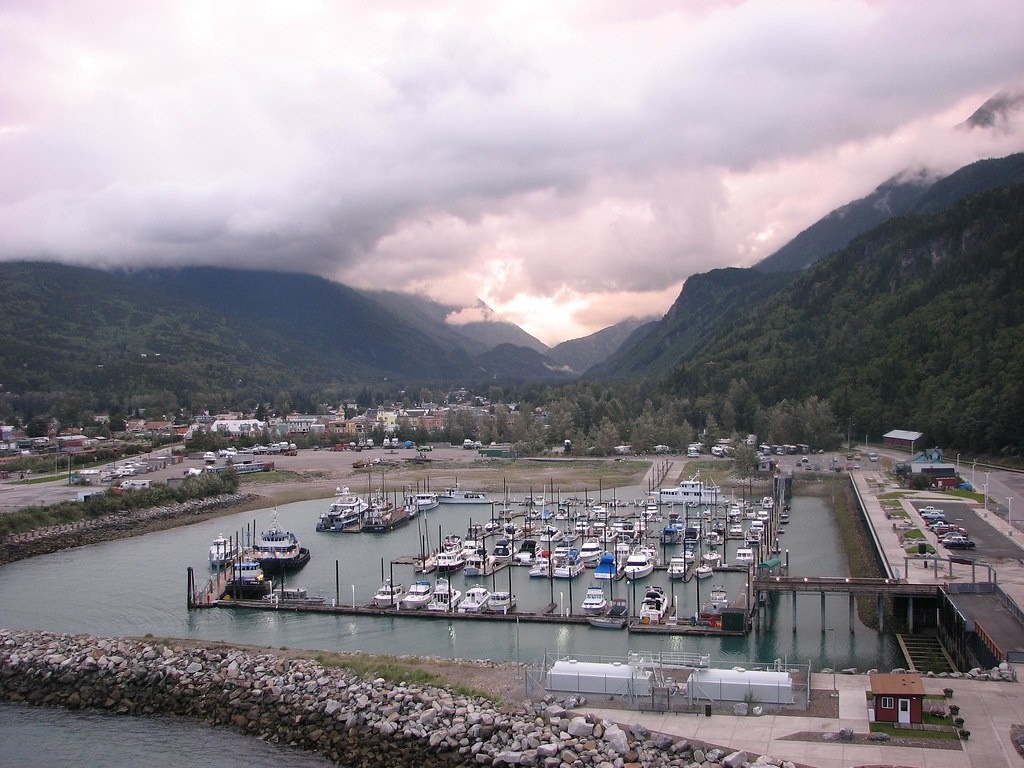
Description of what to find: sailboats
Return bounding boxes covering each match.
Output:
[207,469,791,616]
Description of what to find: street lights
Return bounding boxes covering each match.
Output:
[268,580,273,604]
[972,463,976,492]
[866,434,869,450]
[981,483,989,519]
[983,472,990,500]
[559,591,563,618]
[911,440,915,456]
[827,627,837,696]
[350,584,355,608]
[1005,496,1015,536]
[675,595,678,621]
[957,453,961,471]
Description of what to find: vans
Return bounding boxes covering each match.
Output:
[868,453,878,463]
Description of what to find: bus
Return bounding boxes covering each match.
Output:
[782,444,798,455]
[686,443,784,457]
[796,444,810,454]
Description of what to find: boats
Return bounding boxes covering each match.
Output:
[637,584,669,620]
[585,616,626,629]
[579,579,609,616]
[701,584,732,615]
[604,597,629,618]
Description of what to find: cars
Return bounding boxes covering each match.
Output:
[918,504,977,550]
[795,449,862,473]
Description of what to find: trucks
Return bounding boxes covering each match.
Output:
[116,479,153,491]
[72,454,185,481]
[201,435,483,465]
